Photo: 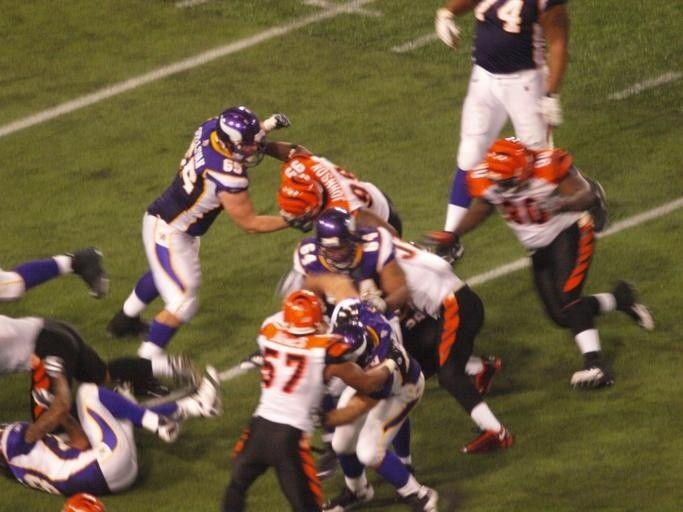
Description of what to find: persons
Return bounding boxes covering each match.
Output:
[314,304,438,509]
[390,235,512,452]
[106,105,292,377]
[418,136,655,390]
[274,206,413,477]
[0,315,202,435]
[0,355,223,497]
[223,289,395,511]
[0,246,110,302]
[237,140,402,239]
[434,0,570,264]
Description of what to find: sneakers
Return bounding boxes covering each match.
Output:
[114,353,224,442]
[464,423,513,453]
[313,443,338,479]
[106,310,149,337]
[71,247,109,298]
[614,280,654,332]
[570,363,613,388]
[473,354,503,399]
[320,481,374,512]
[439,239,464,265]
[415,484,439,512]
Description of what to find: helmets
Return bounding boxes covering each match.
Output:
[332,319,371,363]
[284,289,323,326]
[277,172,324,217]
[216,106,267,169]
[317,206,357,249]
[486,137,534,187]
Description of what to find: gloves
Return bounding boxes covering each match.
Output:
[361,290,387,315]
[44,355,64,377]
[273,113,290,128]
[435,6,462,50]
[31,387,55,410]
[539,95,563,126]
